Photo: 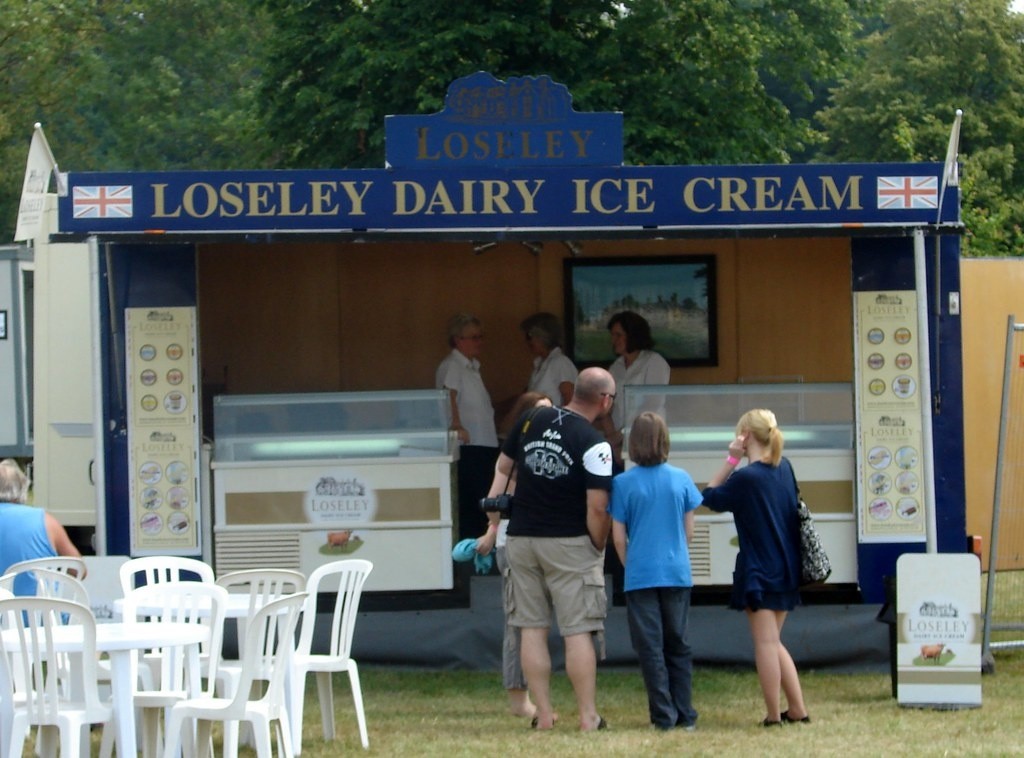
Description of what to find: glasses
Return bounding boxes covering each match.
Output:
[601,391,617,404]
[459,335,482,341]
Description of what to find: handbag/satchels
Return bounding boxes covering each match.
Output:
[798,498,832,587]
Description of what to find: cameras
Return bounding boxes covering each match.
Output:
[481,493,515,520]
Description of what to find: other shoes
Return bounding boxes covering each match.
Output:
[578,718,609,732]
[655,723,696,732]
[758,711,809,727]
[530,714,556,730]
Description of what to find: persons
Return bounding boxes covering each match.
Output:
[475,366,617,731]
[610,411,705,733]
[0,458,87,626]
[435,313,500,447]
[520,312,579,406]
[699,409,816,729]
[606,311,671,427]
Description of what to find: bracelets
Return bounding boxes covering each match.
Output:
[489,524,498,531]
[727,456,739,466]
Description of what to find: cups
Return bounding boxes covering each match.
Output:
[897,379,911,393]
[169,393,181,410]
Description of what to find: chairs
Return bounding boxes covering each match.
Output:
[215,567,308,758]
[118,555,219,738]
[0,555,117,758]
[167,588,312,758]
[260,556,376,747]
[108,581,233,758]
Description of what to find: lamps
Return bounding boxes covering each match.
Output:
[518,238,540,258]
[472,239,497,257]
[561,239,586,255]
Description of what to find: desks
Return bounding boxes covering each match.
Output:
[114,586,308,758]
[0,620,211,758]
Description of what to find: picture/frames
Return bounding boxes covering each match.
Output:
[558,251,721,366]
